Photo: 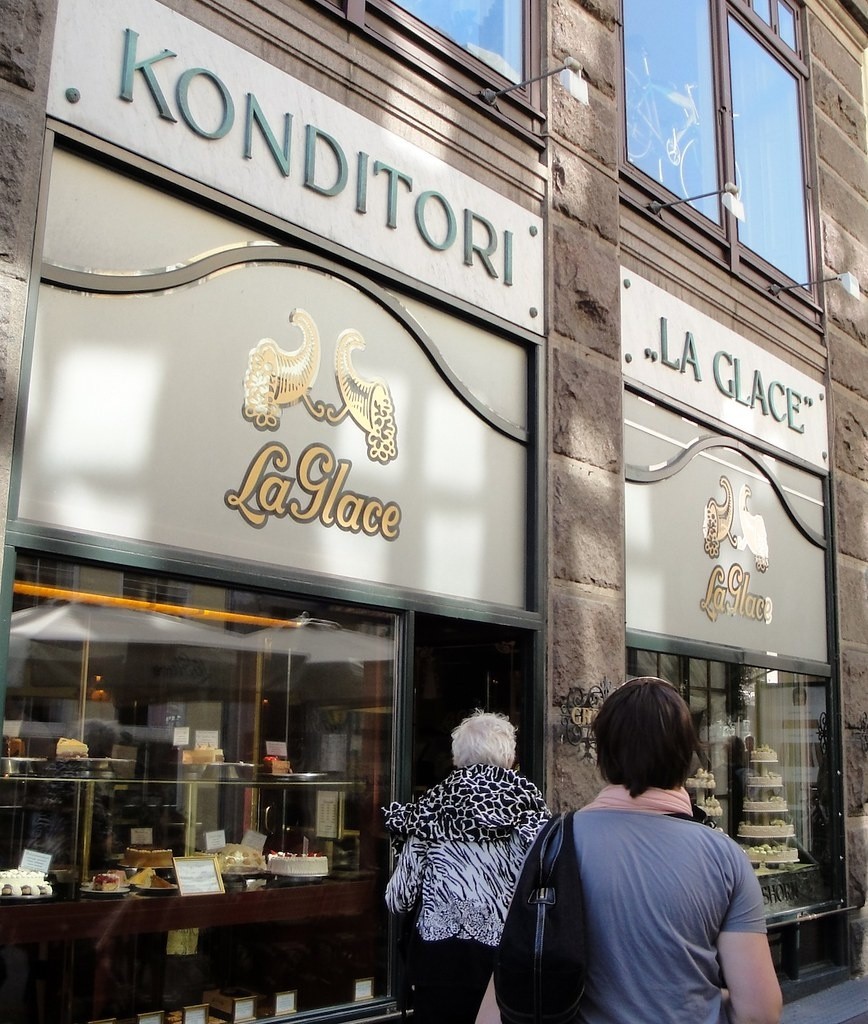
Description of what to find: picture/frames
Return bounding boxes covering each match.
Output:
[136,1010,165,1024]
[352,977,375,1002]
[88,1018,116,1024]
[172,856,224,898]
[232,996,258,1022]
[182,1003,210,1024]
[273,989,298,1016]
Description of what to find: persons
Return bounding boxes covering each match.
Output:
[16,718,194,1024]
[387,708,553,1024]
[472,676,783,1024]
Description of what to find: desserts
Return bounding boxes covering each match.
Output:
[55,737,89,759]
[93,867,174,892]
[191,743,225,763]
[263,756,290,774]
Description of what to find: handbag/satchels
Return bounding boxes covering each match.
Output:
[492,810,591,1024]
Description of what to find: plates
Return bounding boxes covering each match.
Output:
[258,771,337,782]
[79,881,178,892]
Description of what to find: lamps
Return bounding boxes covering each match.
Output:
[766,272,860,303]
[648,181,747,225]
[479,57,590,109]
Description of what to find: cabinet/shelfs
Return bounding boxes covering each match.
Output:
[0,562,392,1024]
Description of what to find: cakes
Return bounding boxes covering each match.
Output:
[268,850,328,875]
[124,844,174,868]
[195,843,267,873]
[0,869,53,897]
